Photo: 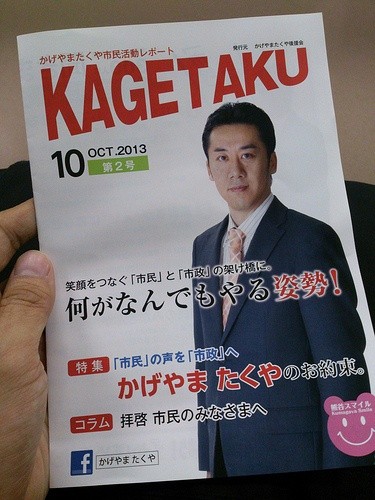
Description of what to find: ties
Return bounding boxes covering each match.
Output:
[221,229,244,331]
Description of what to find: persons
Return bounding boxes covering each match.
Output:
[192,102,371,480]
